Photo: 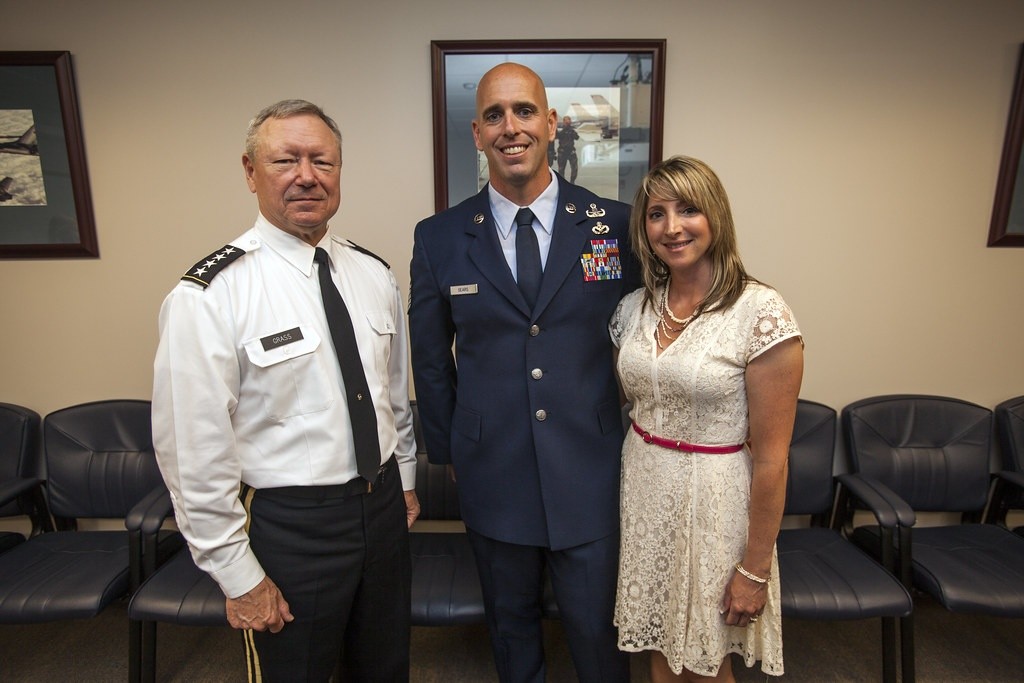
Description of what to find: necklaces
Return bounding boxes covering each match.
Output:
[655,275,699,350]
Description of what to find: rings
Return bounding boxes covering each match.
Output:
[749,617,758,623]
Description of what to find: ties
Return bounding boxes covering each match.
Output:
[514,206,543,312]
[313,245,381,486]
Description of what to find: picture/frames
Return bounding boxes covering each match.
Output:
[986,43,1024,247]
[431,38,667,215]
[0,49,101,259]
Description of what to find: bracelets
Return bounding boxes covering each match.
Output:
[736,565,772,583]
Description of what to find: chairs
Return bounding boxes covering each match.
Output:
[776,397,1023,683]
[0,398,489,683]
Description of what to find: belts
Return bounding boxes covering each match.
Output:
[631,420,745,454]
[258,452,395,499]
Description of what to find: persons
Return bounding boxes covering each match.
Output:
[606,155,804,683]
[556,116,580,184]
[151,99,421,683]
[408,61,643,683]
